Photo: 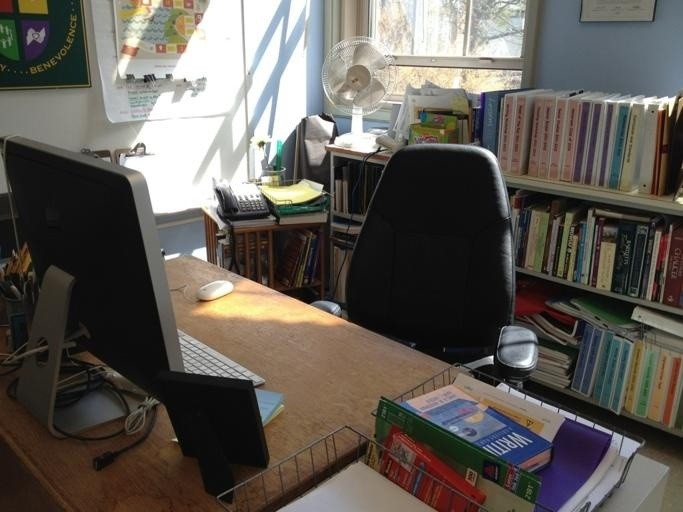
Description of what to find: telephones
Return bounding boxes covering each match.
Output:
[215,187,270,219]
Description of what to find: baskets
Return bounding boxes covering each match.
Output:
[370,362,644,512]
[215,425,486,512]
[216,363,646,508]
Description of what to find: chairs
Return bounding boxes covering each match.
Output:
[308,143,537,390]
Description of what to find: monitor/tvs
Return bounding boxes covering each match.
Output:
[0,134,185,439]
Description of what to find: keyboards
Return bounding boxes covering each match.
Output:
[177,329,266,388]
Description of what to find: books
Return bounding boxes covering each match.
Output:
[398,385,553,474]
[216,160,354,303]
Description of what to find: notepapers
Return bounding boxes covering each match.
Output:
[255,388,285,428]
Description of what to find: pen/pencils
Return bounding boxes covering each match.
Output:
[0,248,21,301]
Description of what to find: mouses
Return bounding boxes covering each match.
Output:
[197,281,233,301]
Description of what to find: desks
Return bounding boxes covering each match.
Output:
[261,382,669,511]
[1,256,473,512]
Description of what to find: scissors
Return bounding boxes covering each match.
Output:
[23,279,39,305]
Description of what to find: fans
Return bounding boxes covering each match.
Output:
[320,36,398,146]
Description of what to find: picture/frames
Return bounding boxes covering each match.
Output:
[577,0,657,23]
[0,0,93,91]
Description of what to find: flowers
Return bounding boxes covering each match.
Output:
[248,132,278,171]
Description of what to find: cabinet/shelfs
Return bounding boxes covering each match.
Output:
[203,207,327,304]
[324,144,393,306]
[500,173,681,442]
